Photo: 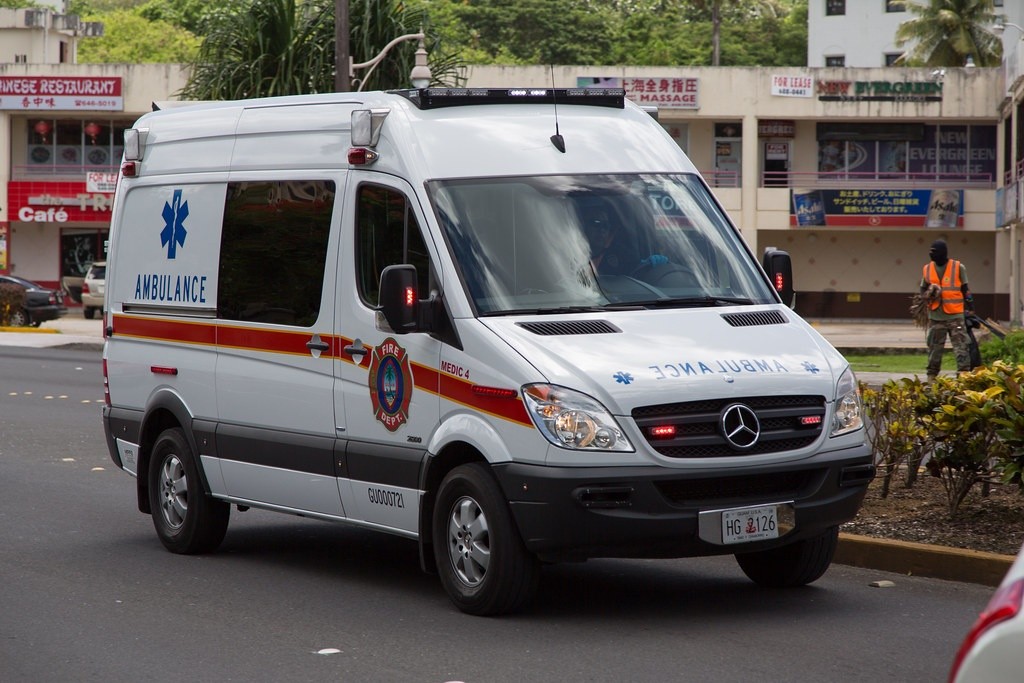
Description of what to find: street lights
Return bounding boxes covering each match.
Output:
[334,27,432,93]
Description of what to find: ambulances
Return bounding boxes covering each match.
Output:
[101,87,878,619]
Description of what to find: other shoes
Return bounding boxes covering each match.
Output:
[927,375,937,385]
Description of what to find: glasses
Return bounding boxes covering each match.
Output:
[584,218,612,230]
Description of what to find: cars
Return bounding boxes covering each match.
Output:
[0,274,69,327]
[81,262,107,319]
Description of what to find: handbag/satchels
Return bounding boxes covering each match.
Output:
[966,321,981,369]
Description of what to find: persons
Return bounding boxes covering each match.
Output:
[919,240,980,381]
[545,208,668,289]
